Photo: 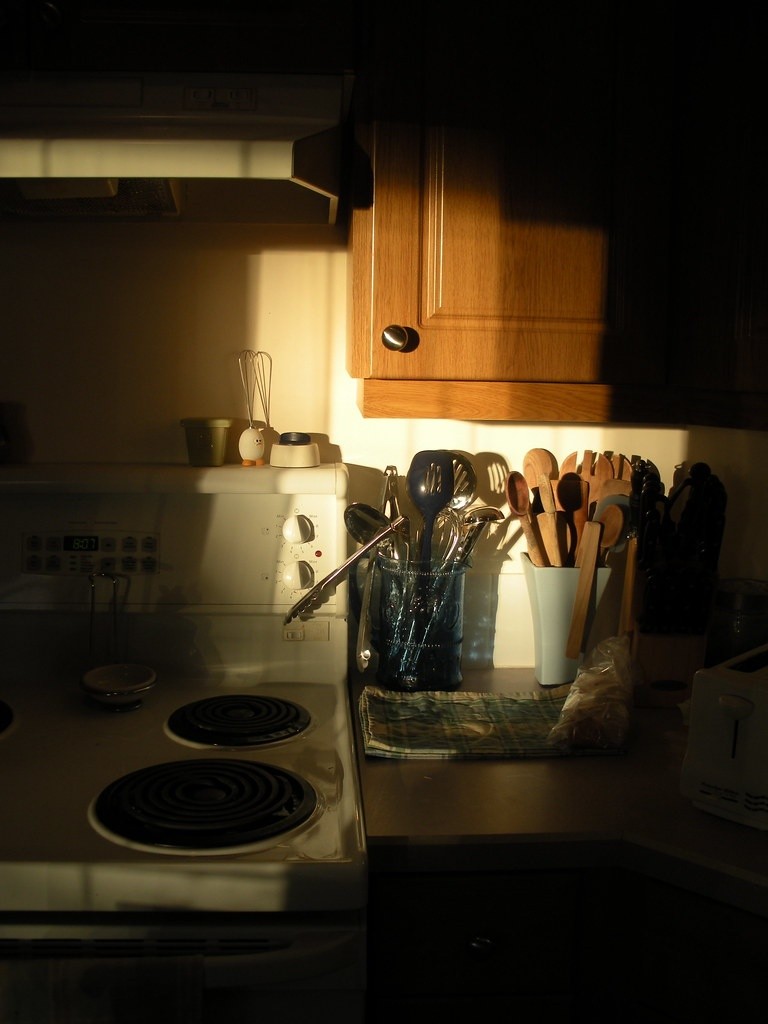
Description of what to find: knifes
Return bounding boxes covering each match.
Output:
[628,455,727,635]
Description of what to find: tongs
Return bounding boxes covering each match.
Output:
[354,463,412,672]
[284,513,412,667]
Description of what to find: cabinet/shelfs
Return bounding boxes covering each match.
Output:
[2,71,354,226]
[338,0,767,436]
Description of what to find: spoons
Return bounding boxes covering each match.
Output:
[594,505,623,568]
[523,447,553,499]
[505,469,546,568]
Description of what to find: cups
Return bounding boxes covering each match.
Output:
[377,556,468,694]
[180,415,234,467]
[518,548,611,687]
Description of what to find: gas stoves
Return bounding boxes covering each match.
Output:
[0,455,372,933]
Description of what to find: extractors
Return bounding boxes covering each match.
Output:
[1,1,356,240]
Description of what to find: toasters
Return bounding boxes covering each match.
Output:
[680,638,767,838]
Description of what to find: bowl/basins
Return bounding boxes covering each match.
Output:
[84,656,157,702]
